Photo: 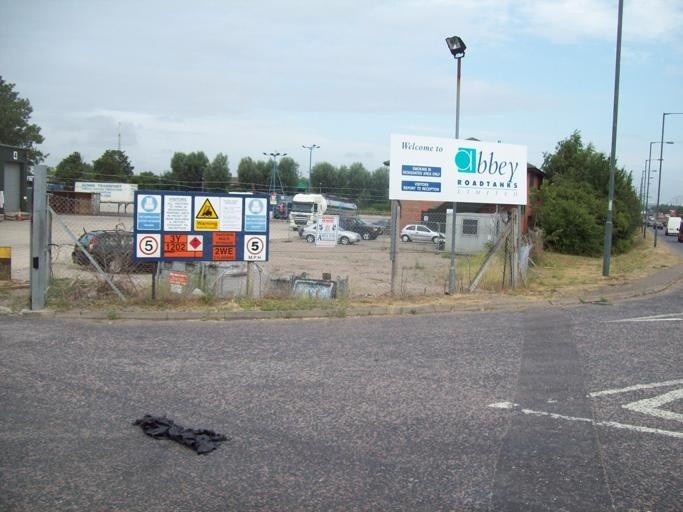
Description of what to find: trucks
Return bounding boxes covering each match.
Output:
[288,193,358,230]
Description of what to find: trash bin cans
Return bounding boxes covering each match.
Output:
[0,246,11,280]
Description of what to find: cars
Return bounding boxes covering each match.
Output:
[338,218,382,239]
[639,208,683,241]
[72,229,155,274]
[399,224,445,244]
[302,226,363,244]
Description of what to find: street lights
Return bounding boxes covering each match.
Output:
[302,144,321,192]
[445,34,467,294]
[639,141,676,247]
[262,153,286,195]
